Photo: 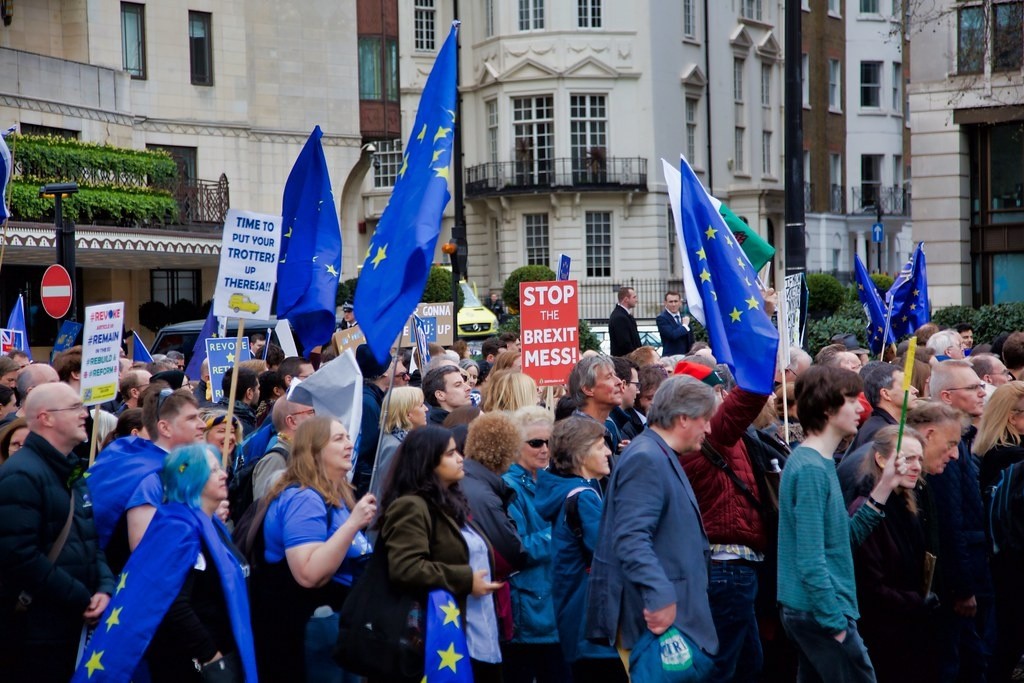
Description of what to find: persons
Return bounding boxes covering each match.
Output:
[486,293,503,326]
[656,291,694,357]
[0,325,1024,683]
[334,299,358,332]
[608,287,643,357]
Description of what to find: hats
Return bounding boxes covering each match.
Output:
[674,361,723,387]
[343,304,354,312]
[150,370,185,390]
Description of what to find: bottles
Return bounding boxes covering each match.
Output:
[768,458,782,473]
[398,600,424,647]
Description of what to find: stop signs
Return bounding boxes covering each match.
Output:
[40,264,73,319]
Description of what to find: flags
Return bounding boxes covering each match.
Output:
[7,294,33,364]
[885,241,930,341]
[184,294,224,380]
[354,22,460,366]
[854,253,896,358]
[276,124,342,363]
[661,152,780,395]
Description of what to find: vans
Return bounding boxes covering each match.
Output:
[149,315,343,362]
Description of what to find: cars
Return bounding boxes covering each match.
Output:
[454,279,500,339]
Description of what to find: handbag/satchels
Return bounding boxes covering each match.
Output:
[629,625,714,683]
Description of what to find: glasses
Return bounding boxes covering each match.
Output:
[9,443,23,451]
[156,388,175,421]
[292,408,315,416]
[626,381,641,390]
[182,381,191,389]
[395,373,411,381]
[37,404,85,420]
[525,438,549,448]
[947,384,986,393]
